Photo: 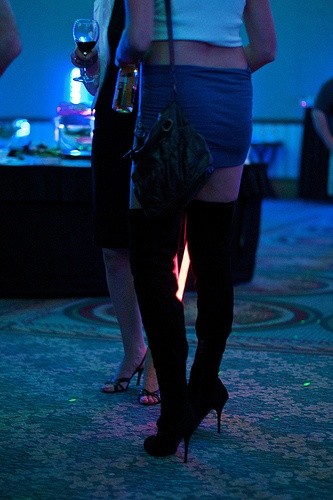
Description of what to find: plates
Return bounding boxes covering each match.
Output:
[60,150,91,159]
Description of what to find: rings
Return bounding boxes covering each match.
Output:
[73,57,78,61]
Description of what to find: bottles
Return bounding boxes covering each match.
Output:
[111,63,140,115]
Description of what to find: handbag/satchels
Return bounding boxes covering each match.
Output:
[121,103,217,223]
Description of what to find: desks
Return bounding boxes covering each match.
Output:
[0,152,269,296]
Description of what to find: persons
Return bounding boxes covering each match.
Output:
[297,78,333,201]
[114,0,276,463]
[70,1,191,406]
[0,0,23,77]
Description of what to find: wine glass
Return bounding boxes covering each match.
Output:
[71,17,101,83]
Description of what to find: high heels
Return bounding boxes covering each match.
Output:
[101,353,147,392]
[144,383,202,462]
[138,388,162,405]
[191,375,229,432]
